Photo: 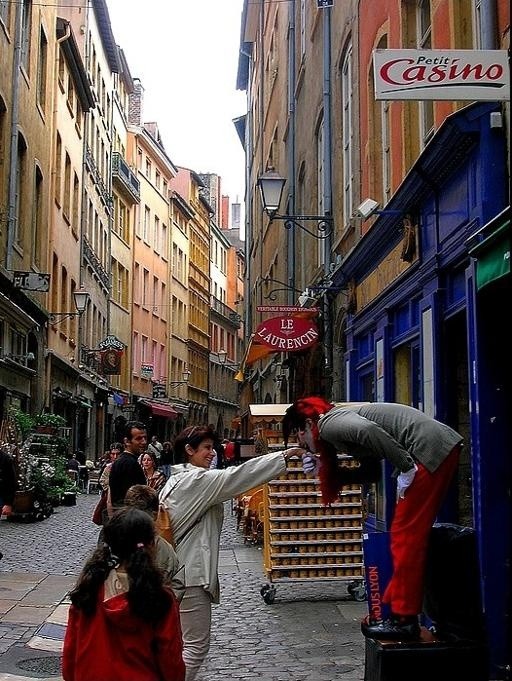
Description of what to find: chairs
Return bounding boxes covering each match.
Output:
[84,459,101,496]
[231,488,264,544]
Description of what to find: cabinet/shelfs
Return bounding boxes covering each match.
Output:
[260,440,368,603]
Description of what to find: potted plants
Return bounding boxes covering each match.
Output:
[0,405,79,521]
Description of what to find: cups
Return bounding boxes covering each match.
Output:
[267,446,365,579]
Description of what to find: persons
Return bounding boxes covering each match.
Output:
[281,397,464,639]
[103,353,118,374]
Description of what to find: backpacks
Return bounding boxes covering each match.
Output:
[151,471,209,548]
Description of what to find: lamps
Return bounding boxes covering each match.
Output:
[254,164,335,238]
[234,333,257,382]
[169,368,191,388]
[217,345,240,366]
[47,283,91,327]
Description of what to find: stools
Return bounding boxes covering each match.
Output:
[362,614,443,681]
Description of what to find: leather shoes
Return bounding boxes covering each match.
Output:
[359,617,422,640]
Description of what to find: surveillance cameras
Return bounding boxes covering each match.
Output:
[355,198,380,222]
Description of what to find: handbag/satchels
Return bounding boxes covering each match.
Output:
[91,493,112,525]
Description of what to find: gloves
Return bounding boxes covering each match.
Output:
[394,464,418,500]
[301,454,324,476]
